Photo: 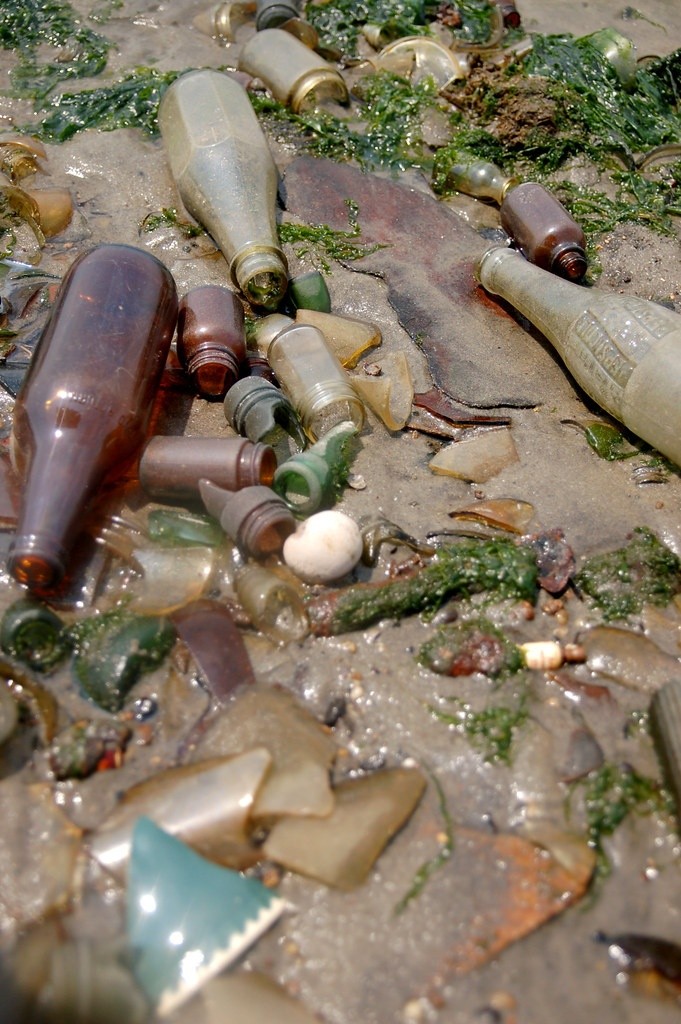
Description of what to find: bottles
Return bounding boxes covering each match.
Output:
[448,158,515,205]
[181,283,240,400]
[265,324,367,440]
[255,0,302,33]
[135,432,278,489]
[501,181,587,280]
[0,569,65,672]
[14,241,181,596]
[474,245,681,471]
[155,69,293,311]
[240,29,351,124]
[190,0,244,45]
[223,375,303,454]
